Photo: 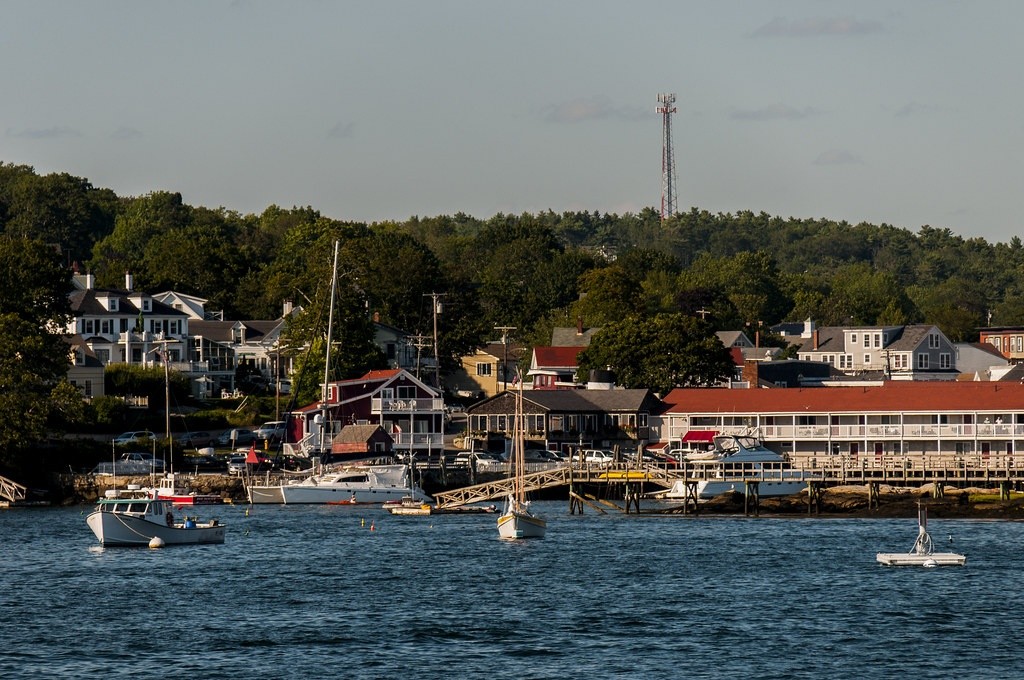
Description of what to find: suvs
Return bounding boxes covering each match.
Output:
[119,452,167,471]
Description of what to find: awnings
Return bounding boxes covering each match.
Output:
[683,431,719,443]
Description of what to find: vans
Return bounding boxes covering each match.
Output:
[116,431,157,445]
[572,450,613,463]
[258,421,286,440]
[453,451,499,464]
[526,450,570,463]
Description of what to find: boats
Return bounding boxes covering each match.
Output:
[246,458,434,504]
[86,485,226,546]
[667,434,808,496]
[382,495,431,517]
[876,498,966,568]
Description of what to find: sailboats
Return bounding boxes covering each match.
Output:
[497,379,545,539]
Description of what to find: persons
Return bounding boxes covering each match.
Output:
[166,514,172,526]
[984,417,991,424]
[996,417,1003,424]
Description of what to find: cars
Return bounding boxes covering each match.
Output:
[218,429,258,445]
[227,456,247,475]
